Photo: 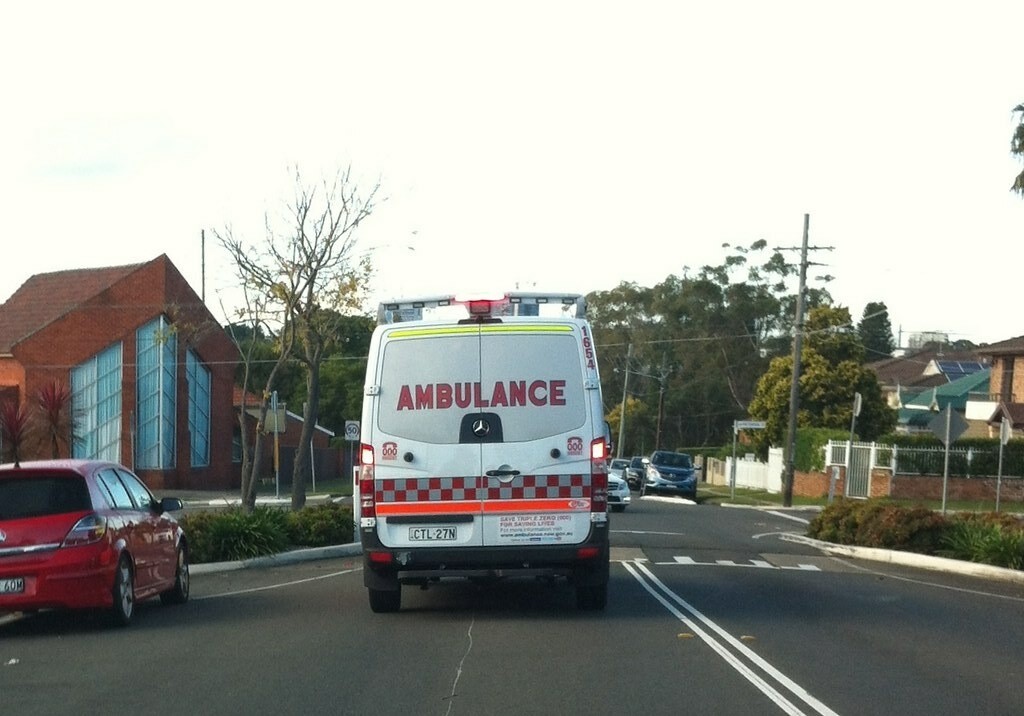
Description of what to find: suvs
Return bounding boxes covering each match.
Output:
[608,451,703,512]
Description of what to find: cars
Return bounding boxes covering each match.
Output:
[0,459,190,627]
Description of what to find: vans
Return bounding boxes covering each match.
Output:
[353,293,611,614]
